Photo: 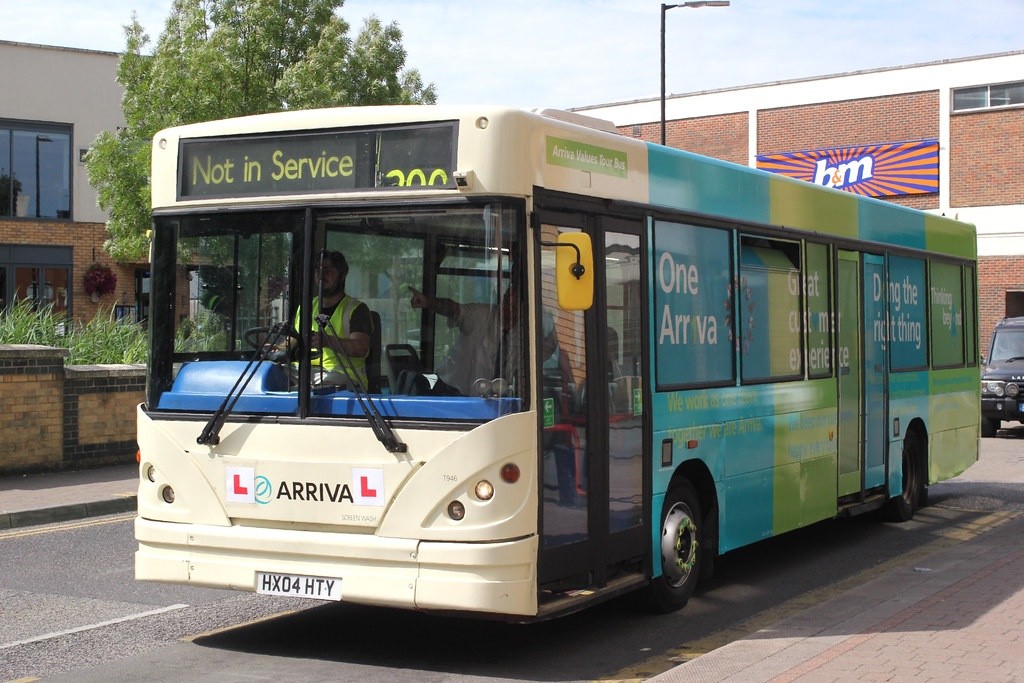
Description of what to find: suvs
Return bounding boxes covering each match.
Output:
[980,313,1024,439]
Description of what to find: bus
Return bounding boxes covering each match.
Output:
[136,102,982,620]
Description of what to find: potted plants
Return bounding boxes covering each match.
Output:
[84,263,117,302]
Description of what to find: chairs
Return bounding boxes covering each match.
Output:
[364,310,382,394]
[606,326,622,376]
[383,344,424,396]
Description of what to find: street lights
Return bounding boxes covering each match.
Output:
[35,134,53,219]
[660,0,732,148]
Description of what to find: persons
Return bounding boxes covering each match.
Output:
[407,275,558,396]
[540,441,587,509]
[264,251,372,391]
